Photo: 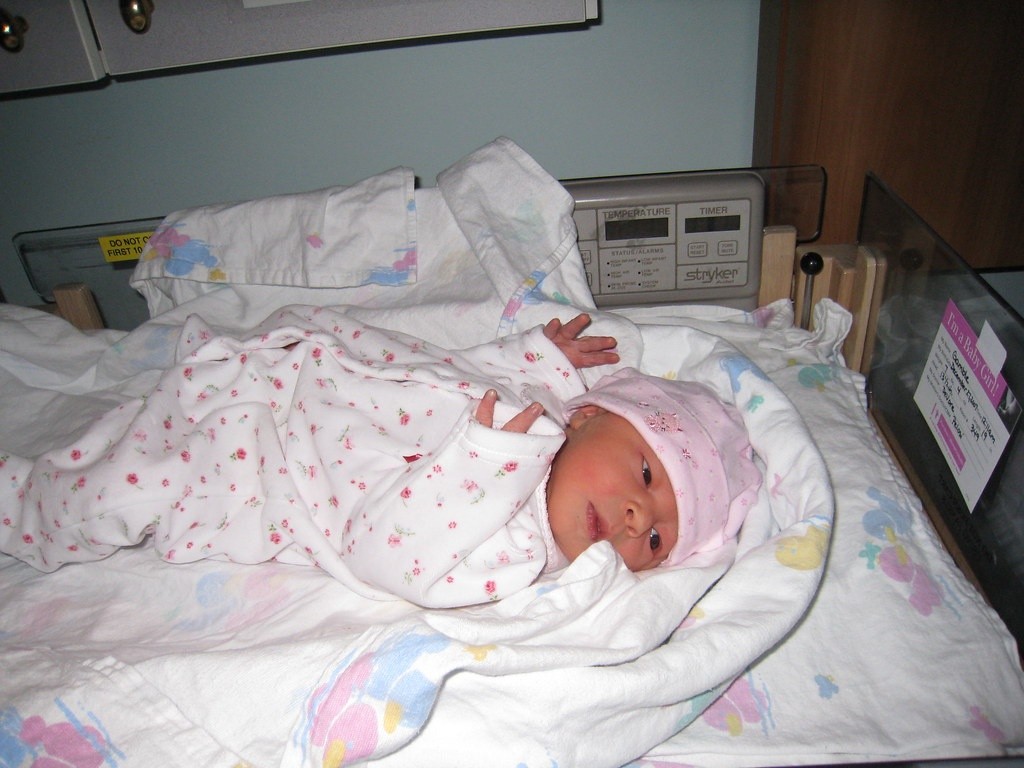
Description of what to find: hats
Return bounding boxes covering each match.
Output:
[563,364,766,571]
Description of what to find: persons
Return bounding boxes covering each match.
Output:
[0,309,764,610]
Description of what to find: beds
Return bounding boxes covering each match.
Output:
[0,303,1024,768]
